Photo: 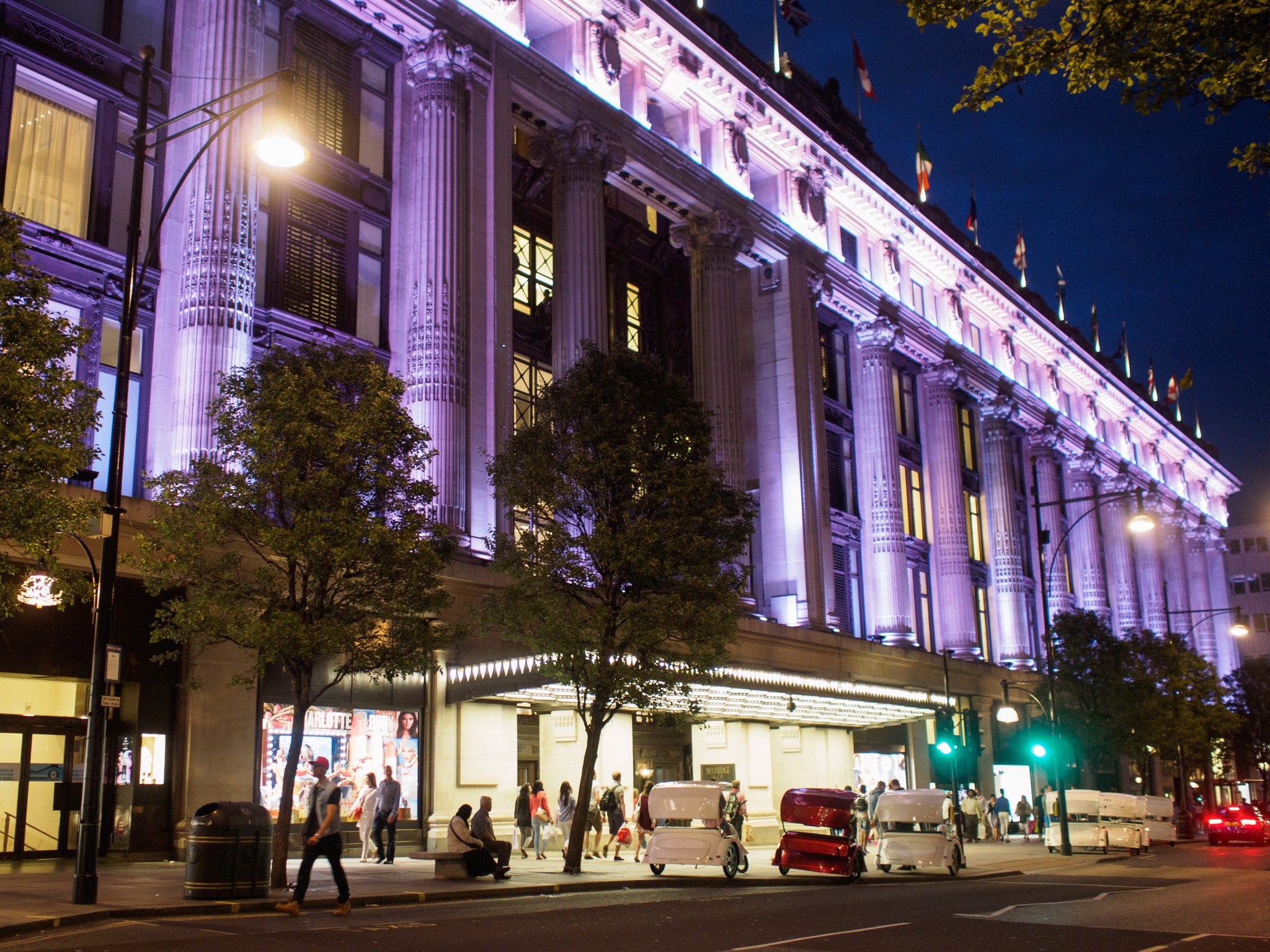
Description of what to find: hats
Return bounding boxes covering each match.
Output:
[309,756,329,770]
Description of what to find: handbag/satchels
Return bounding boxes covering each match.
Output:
[542,823,558,846]
[352,807,362,820]
[466,832,479,848]
[745,821,754,841]
[302,810,319,837]
[633,793,643,821]
[512,828,519,850]
[614,822,633,849]
[534,791,550,823]
[977,820,986,839]
[1008,815,1039,833]
[513,817,518,828]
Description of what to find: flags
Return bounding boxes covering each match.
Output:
[779,0,1194,406]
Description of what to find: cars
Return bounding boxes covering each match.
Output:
[1203,805,1270,847]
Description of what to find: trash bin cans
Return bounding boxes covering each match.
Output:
[182,801,274,900]
[1177,810,1195,840]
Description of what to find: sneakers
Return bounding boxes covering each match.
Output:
[276,901,300,917]
[334,901,351,915]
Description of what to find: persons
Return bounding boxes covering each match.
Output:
[1175,797,1270,836]
[600,771,628,861]
[448,804,511,881]
[117,738,133,786]
[557,781,576,860]
[632,781,667,862]
[701,779,748,842]
[514,782,533,858]
[276,711,419,783]
[529,780,555,860]
[348,766,401,864]
[584,769,602,859]
[274,757,352,918]
[845,779,1127,871]
[470,796,512,879]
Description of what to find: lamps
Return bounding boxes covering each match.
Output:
[786,696,795,712]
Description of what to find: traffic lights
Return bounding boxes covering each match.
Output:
[1196,795,1203,802]
[937,735,956,756]
[1032,743,1047,759]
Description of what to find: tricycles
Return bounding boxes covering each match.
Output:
[771,787,870,884]
[1044,787,1178,856]
[640,779,749,880]
[873,788,965,878]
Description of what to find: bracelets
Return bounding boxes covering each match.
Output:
[315,833,321,839]
[603,815,605,817]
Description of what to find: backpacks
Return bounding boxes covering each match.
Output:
[856,793,868,811]
[725,791,740,814]
[600,785,622,811]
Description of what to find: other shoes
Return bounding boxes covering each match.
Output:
[583,845,641,862]
[494,874,512,880]
[868,836,878,846]
[520,847,547,860]
[863,848,870,854]
[562,848,567,859]
[1050,848,1060,852]
[966,835,1045,846]
[360,846,396,862]
[496,866,510,875]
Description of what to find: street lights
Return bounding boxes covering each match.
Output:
[994,456,1154,856]
[1162,579,1251,841]
[14,47,313,902]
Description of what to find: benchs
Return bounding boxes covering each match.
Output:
[655,824,735,839]
[1049,819,1171,828]
[409,851,498,879]
[787,831,858,853]
[884,829,957,842]
